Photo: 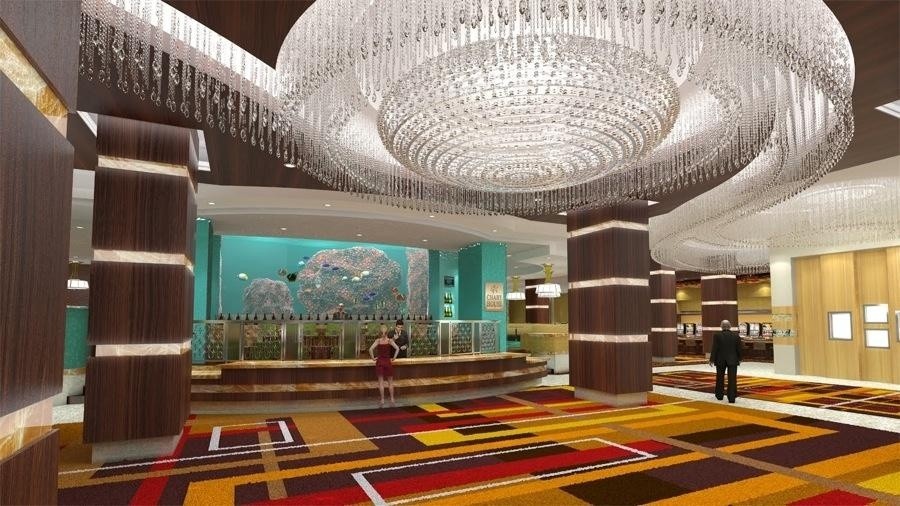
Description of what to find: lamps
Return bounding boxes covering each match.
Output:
[506,276,526,301]
[535,263,562,298]
[77,0,900,275]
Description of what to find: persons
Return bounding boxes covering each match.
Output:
[368,324,401,406]
[332,302,349,320]
[387,319,410,359]
[709,319,744,405]
